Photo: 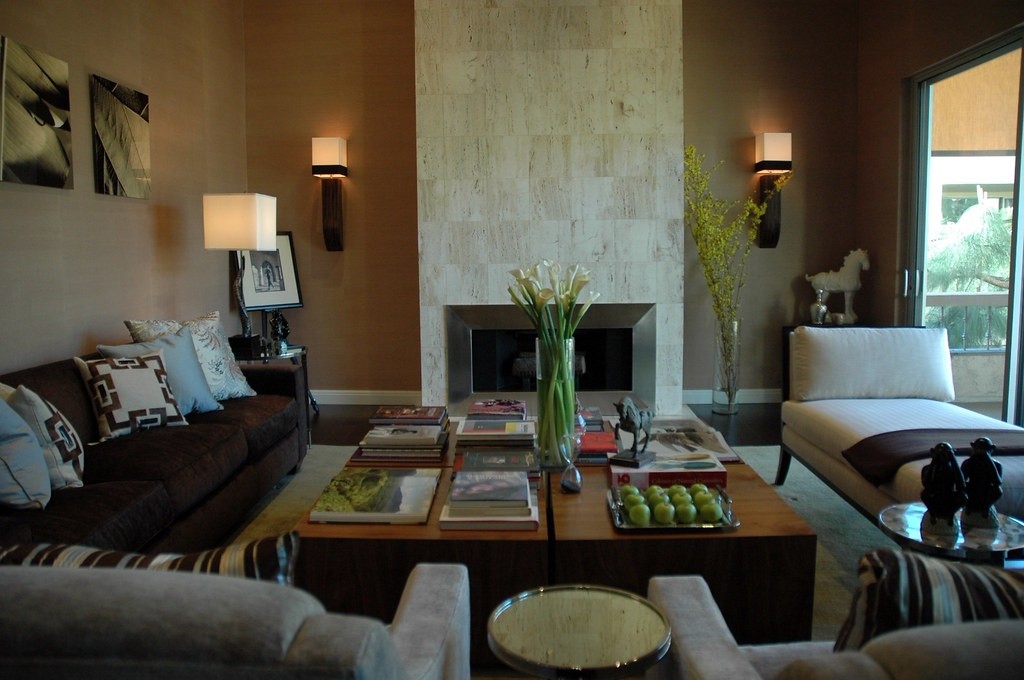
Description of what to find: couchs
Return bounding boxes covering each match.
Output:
[650,573,1024,680]
[0,562,471,680]
[0,352,315,553]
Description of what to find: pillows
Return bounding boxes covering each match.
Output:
[834,548,1024,651]
[1,530,301,588]
[792,326,957,406]
[1,310,259,509]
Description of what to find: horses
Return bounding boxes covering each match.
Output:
[805,248,871,325]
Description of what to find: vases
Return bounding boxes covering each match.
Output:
[711,317,743,417]
[535,336,576,473]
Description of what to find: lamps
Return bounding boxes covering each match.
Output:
[201,190,279,363]
[755,129,792,252]
[311,134,348,255]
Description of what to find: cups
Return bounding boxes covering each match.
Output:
[832,313,845,325]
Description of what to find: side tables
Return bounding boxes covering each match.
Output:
[878,500,1024,588]
[486,585,673,680]
[264,344,321,415]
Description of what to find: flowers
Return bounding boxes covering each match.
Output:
[506,261,601,338]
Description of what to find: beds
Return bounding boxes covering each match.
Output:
[776,325,1024,548]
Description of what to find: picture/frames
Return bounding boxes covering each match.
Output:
[232,231,304,311]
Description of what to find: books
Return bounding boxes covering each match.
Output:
[308,406,451,527]
[440,399,543,531]
[543,407,740,488]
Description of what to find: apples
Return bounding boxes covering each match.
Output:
[621,484,723,526]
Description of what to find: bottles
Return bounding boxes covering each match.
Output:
[811,289,827,324]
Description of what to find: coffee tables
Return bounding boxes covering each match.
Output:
[293,417,822,643]
